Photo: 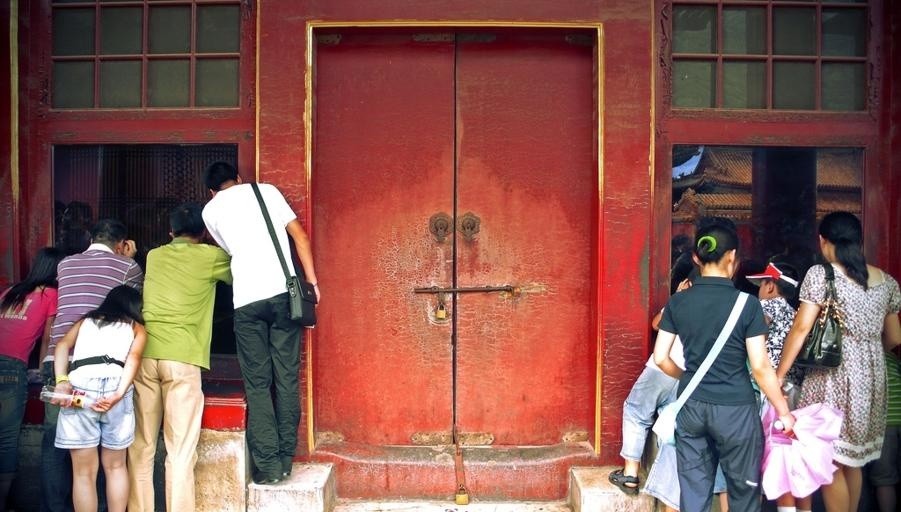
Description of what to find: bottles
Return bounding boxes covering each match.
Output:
[41,385,100,408]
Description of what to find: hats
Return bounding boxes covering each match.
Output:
[743,261,801,288]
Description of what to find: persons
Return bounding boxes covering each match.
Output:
[54,192,184,274]
[40,217,143,511]
[666,216,817,310]
[742,258,815,511]
[608,266,730,512]
[774,210,899,512]
[48,285,149,512]
[125,200,231,512]
[653,221,795,512]
[0,246,63,504]
[199,160,322,484]
[869,351,901,511]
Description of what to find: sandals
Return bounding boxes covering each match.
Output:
[608,467,641,497]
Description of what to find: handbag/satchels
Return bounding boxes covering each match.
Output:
[285,274,319,328]
[792,303,844,370]
[651,401,680,449]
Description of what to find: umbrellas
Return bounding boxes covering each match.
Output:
[759,401,843,502]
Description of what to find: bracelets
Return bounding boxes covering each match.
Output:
[313,282,318,287]
[54,375,67,384]
[776,412,790,418]
